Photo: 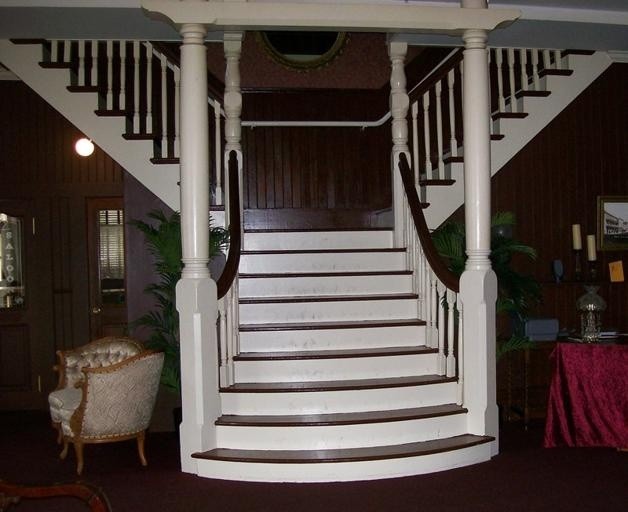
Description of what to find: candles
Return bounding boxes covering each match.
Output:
[586,234,596,261]
[571,223,581,250]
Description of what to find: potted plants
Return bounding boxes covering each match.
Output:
[125,204,231,442]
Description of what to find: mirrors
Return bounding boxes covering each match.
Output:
[257,29,346,73]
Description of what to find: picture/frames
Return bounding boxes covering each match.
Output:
[595,195,627,251]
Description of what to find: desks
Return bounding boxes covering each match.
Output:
[497,334,626,449]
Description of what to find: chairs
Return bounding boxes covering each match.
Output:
[48,334,166,477]
